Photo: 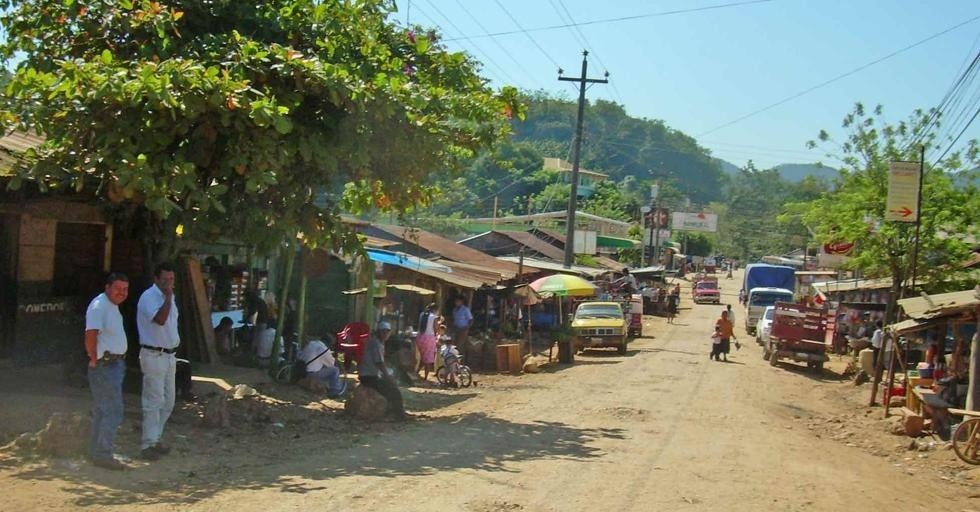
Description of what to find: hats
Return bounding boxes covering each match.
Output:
[378,321,391,330]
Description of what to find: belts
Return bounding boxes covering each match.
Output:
[111,354,127,360]
[143,345,177,353]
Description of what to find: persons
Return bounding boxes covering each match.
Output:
[725,303,735,325]
[716,311,736,361]
[84,272,131,469]
[136,263,180,459]
[610,267,637,298]
[674,282,681,294]
[204,256,475,396]
[358,321,417,422]
[666,290,680,323]
[872,320,883,367]
[709,327,722,360]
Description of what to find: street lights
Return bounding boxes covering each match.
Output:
[640,205,651,269]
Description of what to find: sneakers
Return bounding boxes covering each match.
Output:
[142,443,170,460]
[337,382,347,395]
[95,458,123,470]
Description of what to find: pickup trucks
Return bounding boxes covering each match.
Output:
[690,275,722,305]
[566,301,634,356]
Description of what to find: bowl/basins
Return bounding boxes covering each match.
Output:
[918,368,934,378]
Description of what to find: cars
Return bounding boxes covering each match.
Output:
[754,305,800,349]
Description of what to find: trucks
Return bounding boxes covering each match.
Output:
[742,262,797,334]
[762,299,830,370]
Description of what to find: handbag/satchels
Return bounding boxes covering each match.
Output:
[269,359,305,384]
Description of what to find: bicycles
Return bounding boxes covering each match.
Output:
[952,415,980,468]
[277,350,348,400]
[435,352,473,386]
